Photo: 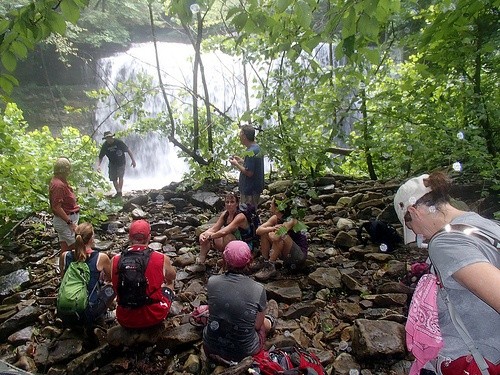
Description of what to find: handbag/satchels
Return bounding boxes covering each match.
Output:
[403,272,443,375]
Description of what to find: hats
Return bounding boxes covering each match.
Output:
[223,240,251,267]
[129,219,151,240]
[102,131,115,140]
[394,174,432,245]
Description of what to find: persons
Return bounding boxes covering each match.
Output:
[202,241,278,360]
[97,132,136,198]
[49,157,80,247]
[60,222,111,325]
[255,193,308,280]
[110,220,176,331]
[185,193,253,274]
[393,170,500,375]
[229,125,264,212]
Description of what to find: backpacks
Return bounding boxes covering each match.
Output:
[118,248,154,309]
[57,261,90,321]
[356,221,399,253]
[239,202,261,240]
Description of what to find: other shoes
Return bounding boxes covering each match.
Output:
[249,256,266,270]
[185,263,206,273]
[268,299,278,324]
[112,192,122,198]
[254,262,277,282]
